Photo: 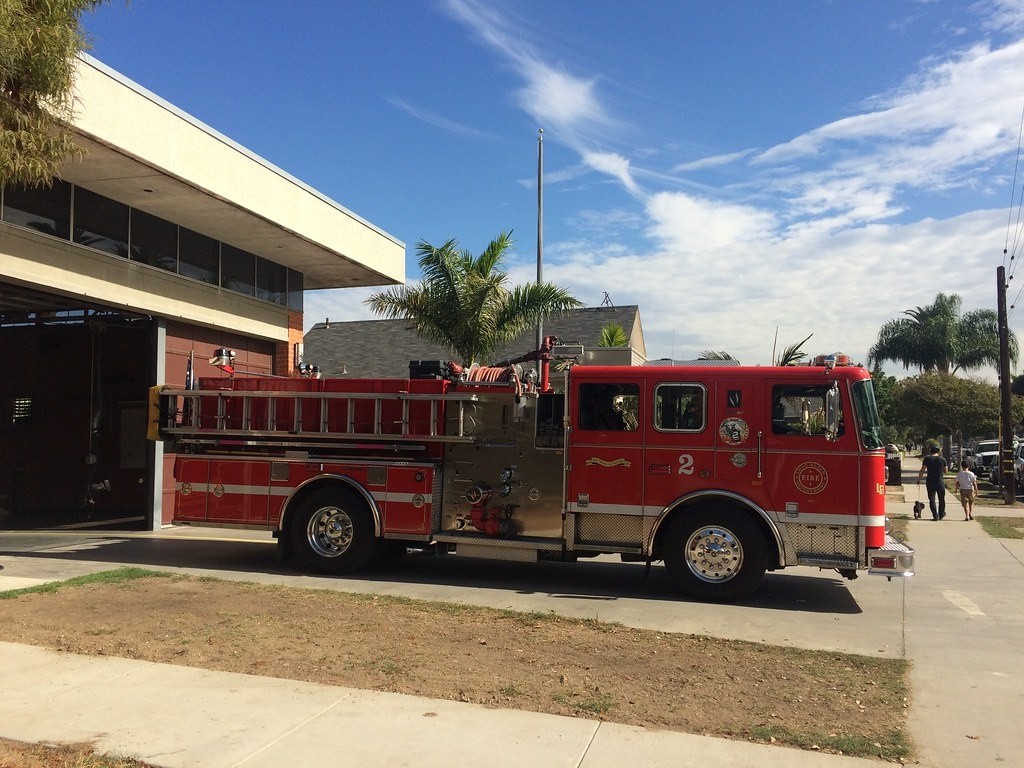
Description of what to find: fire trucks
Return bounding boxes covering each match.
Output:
[144,336,917,603]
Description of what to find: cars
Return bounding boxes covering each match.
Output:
[951,439,1024,488]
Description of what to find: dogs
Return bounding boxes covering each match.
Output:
[913,501,925,519]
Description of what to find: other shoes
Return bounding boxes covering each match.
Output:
[939,512,946,520]
[931,517,938,521]
[969,517,974,520]
[964,518,968,521]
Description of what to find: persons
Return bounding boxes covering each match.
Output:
[954,460,978,521]
[919,446,948,521]
